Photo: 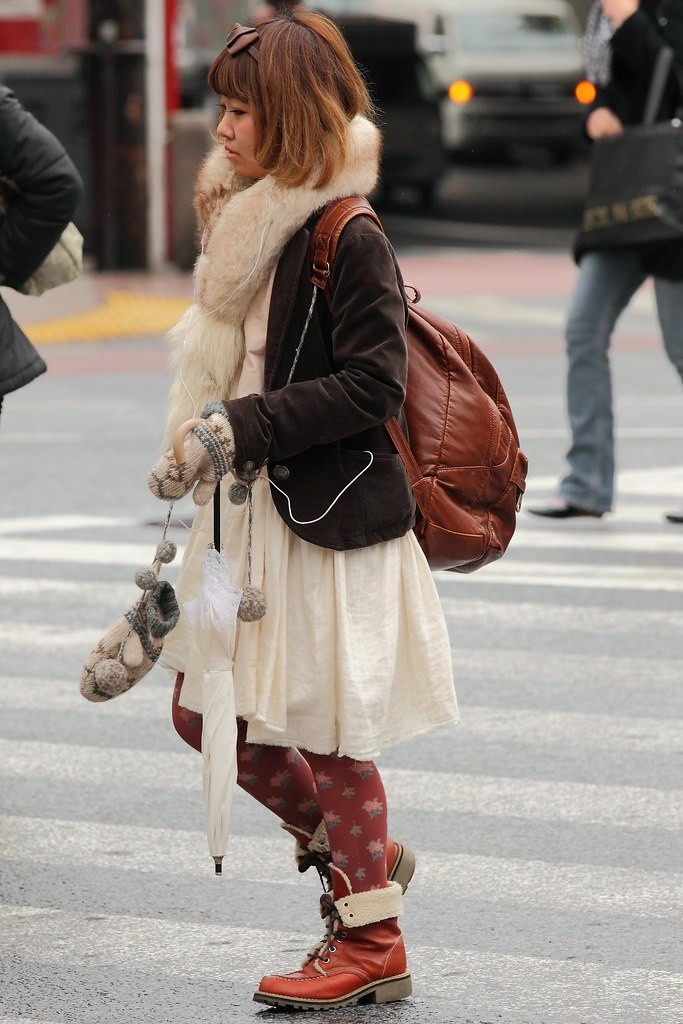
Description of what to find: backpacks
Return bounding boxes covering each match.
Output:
[310,192,528,574]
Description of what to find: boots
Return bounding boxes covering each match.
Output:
[280,817,416,895]
[253,862,412,1010]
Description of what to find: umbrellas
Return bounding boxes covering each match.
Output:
[171,417,241,876]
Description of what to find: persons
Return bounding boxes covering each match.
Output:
[0,83,86,429]
[157,12,460,1007]
[525,0,683,523]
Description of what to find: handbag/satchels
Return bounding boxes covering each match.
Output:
[16,219,85,300]
[572,122,683,268]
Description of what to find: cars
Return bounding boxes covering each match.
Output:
[432,3,595,149]
[297,11,455,214]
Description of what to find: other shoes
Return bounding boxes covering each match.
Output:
[524,505,618,533]
[666,514,683,524]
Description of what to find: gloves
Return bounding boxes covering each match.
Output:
[145,400,237,506]
[79,580,180,701]
